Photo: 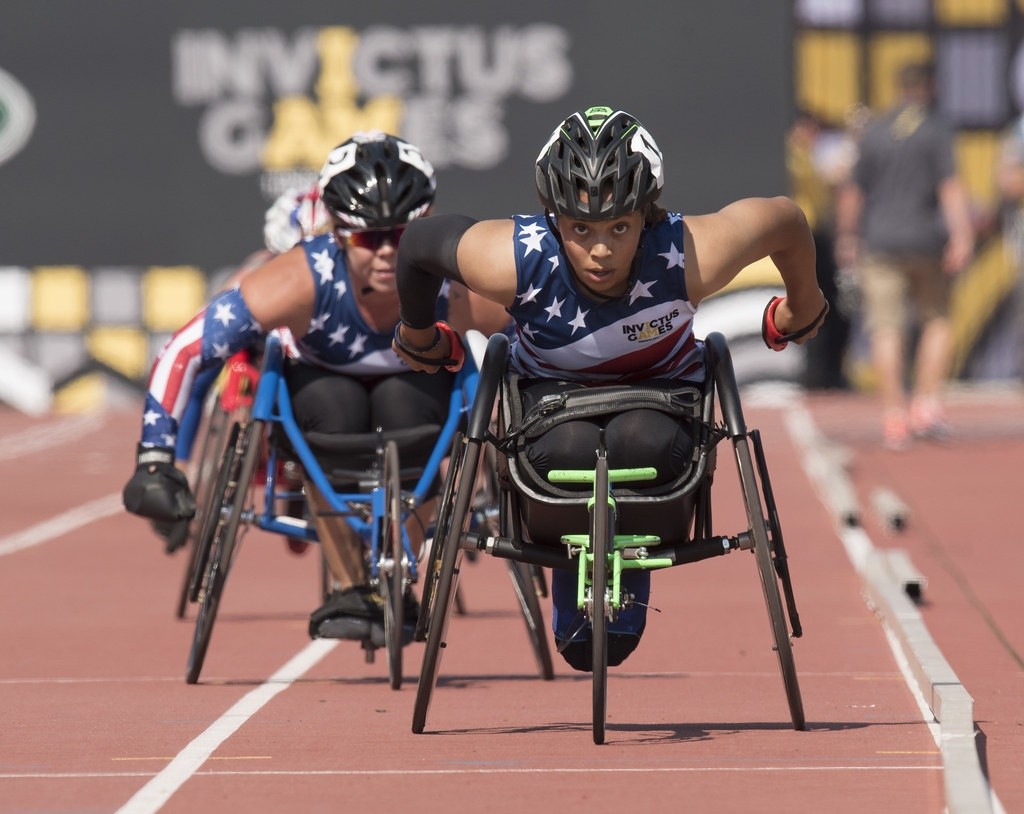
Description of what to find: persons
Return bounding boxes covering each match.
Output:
[783,59,1024,450]
[123,134,521,650]
[391,104,831,673]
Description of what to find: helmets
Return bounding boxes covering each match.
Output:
[317,132,437,232]
[264,189,302,254]
[534,107,665,222]
[297,178,330,242]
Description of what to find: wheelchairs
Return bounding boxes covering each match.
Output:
[160,328,560,683]
[403,331,808,750]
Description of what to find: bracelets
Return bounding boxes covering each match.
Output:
[394,319,441,354]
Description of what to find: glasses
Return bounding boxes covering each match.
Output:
[338,225,406,250]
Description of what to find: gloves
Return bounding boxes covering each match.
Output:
[124,462,195,521]
[153,519,190,554]
